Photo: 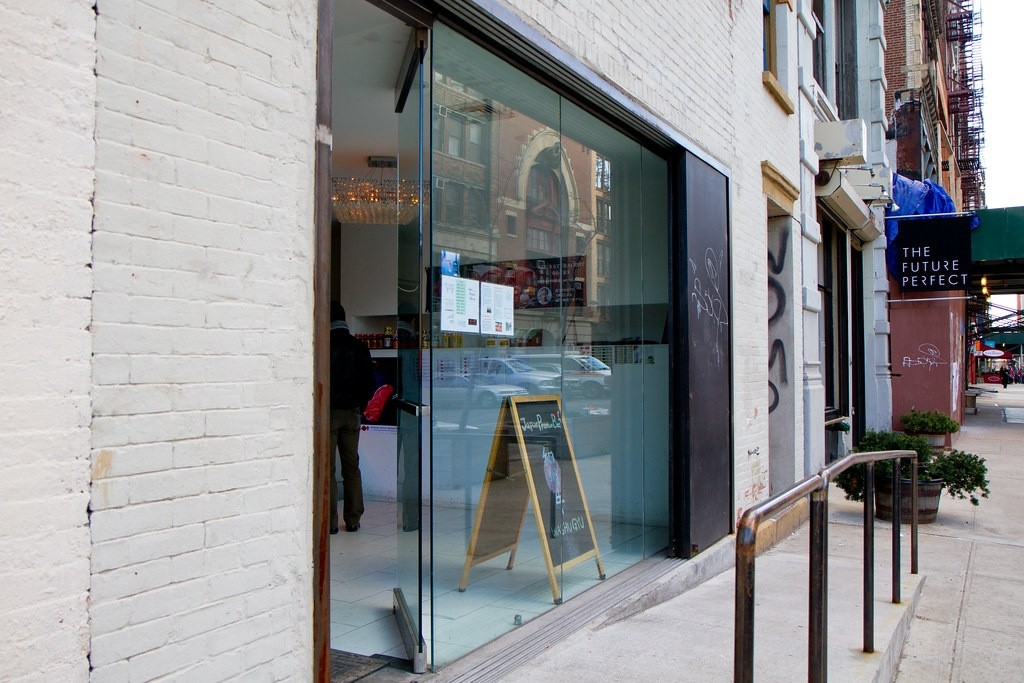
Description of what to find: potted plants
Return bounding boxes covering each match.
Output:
[829,429,992,526]
[902,408,960,456]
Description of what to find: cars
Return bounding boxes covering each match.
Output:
[474,357,563,394]
[432,373,528,408]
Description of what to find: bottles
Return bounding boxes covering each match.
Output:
[355,327,397,349]
[422,330,430,348]
[433,331,462,348]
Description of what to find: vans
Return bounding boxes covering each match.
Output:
[508,349,611,399]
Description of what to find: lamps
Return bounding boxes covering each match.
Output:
[836,162,900,212]
[332,155,428,226]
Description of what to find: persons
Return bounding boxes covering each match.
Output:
[1000,366,1022,388]
[538,289,548,305]
[329,302,375,535]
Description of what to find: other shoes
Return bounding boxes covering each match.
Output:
[330,527,339,534]
[346,522,360,532]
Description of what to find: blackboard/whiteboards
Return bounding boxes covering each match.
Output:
[465,393,599,572]
